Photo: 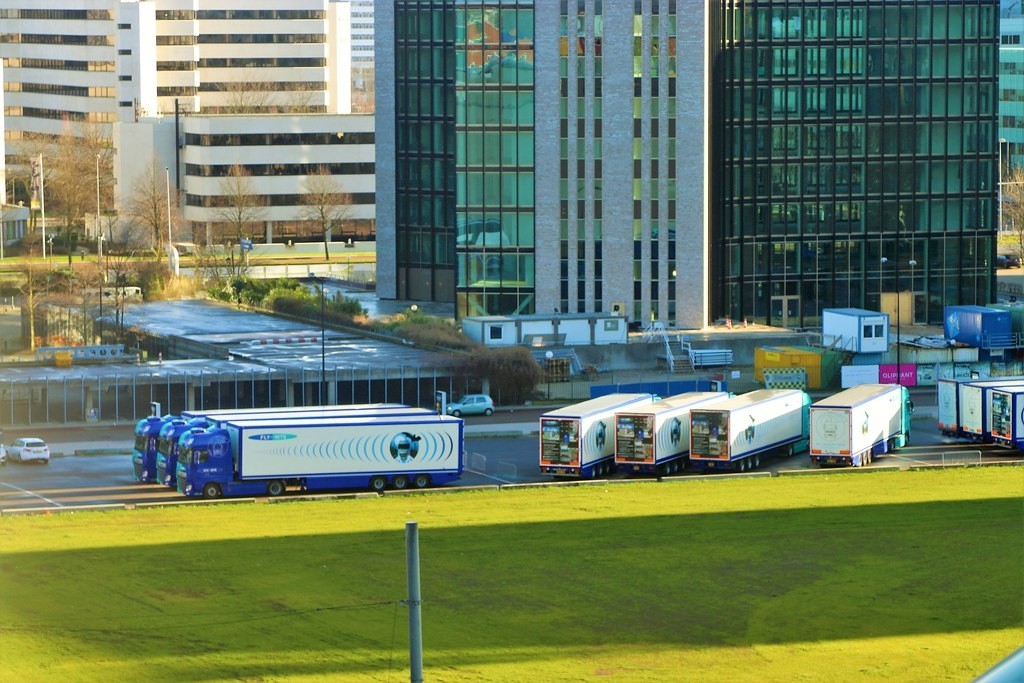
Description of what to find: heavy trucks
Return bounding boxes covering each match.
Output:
[807,383,915,467]
[936,374,1024,454]
[131,401,467,500]
[537,392,664,480]
[612,391,739,477]
[689,387,814,473]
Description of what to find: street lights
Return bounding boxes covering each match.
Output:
[95,154,101,263]
[880,256,918,386]
[998,138,1006,241]
[164,167,171,248]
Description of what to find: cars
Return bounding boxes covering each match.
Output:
[997,254,1022,269]
[446,393,495,416]
[8,437,50,464]
